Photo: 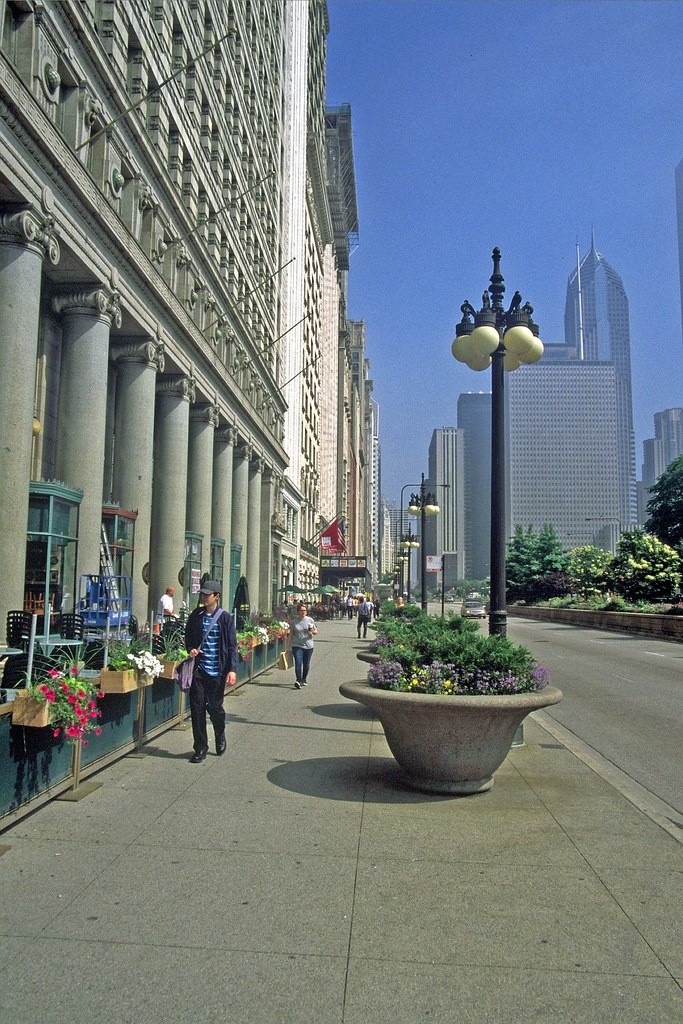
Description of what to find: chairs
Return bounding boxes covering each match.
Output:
[7,608,42,655]
[1,652,59,687]
[59,611,87,639]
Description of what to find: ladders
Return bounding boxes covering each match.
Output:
[99,523,123,612]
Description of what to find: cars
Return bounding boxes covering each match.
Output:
[444,595,454,603]
[460,601,487,619]
[402,591,418,604]
[463,592,490,605]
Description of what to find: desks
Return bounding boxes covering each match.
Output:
[50,611,62,629]
[35,637,84,647]
[0,646,24,656]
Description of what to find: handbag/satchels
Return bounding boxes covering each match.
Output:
[365,616,371,622]
[279,651,294,671]
[175,655,195,691]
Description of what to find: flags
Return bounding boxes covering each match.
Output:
[329,520,346,554]
[319,516,339,549]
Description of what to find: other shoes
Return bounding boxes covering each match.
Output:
[294,681,301,689]
[301,679,307,686]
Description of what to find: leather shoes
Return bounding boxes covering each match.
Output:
[191,749,206,762]
[215,733,226,755]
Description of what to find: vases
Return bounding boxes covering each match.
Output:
[100,666,156,695]
[156,653,191,680]
[11,685,65,728]
[337,617,562,798]
[245,634,262,647]
[280,627,289,635]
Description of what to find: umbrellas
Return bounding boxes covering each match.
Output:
[278,584,341,602]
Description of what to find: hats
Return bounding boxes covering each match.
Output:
[199,580,221,595]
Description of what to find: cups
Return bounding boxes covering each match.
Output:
[308,624,313,631]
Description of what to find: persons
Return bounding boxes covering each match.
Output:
[186,582,238,763]
[328,594,381,621]
[156,585,177,638]
[394,597,404,610]
[356,596,371,639]
[286,603,318,689]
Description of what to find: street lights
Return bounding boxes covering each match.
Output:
[396,548,409,599]
[451,246,544,639]
[399,484,450,597]
[407,472,440,616]
[586,517,622,540]
[567,533,595,548]
[400,521,420,604]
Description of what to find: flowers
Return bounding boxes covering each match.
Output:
[154,625,190,663]
[372,599,552,693]
[106,630,167,679]
[233,619,291,661]
[11,634,109,750]
